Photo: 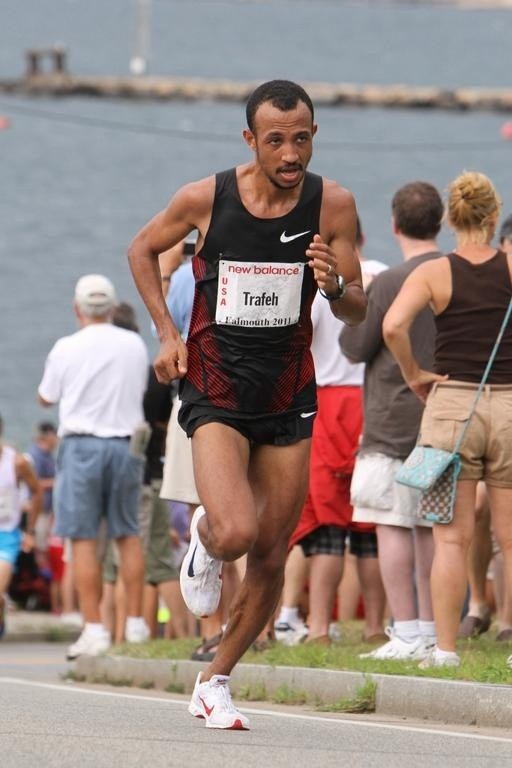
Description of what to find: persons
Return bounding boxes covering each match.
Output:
[270,536,310,648]
[1,418,43,638]
[298,211,389,648]
[60,536,75,618]
[336,182,438,662]
[383,174,510,670]
[97,303,191,641]
[22,420,56,610]
[460,477,503,637]
[39,274,150,653]
[150,239,242,665]
[45,512,61,615]
[127,80,369,730]
[139,585,158,644]
[457,214,512,640]
[328,531,361,646]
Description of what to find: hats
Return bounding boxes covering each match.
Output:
[74,274,117,318]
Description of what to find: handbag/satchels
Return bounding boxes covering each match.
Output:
[394,304,512,523]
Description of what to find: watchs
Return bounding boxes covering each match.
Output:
[318,273,345,302]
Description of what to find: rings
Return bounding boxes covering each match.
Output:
[326,264,331,274]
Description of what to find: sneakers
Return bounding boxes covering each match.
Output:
[418,655,463,669]
[360,636,422,661]
[126,627,154,647]
[459,613,491,638]
[356,634,390,652]
[494,627,511,641]
[418,633,438,656]
[299,634,332,648]
[189,671,255,732]
[65,633,108,661]
[275,621,310,645]
[180,506,227,618]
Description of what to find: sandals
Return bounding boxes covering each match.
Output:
[192,634,225,662]
